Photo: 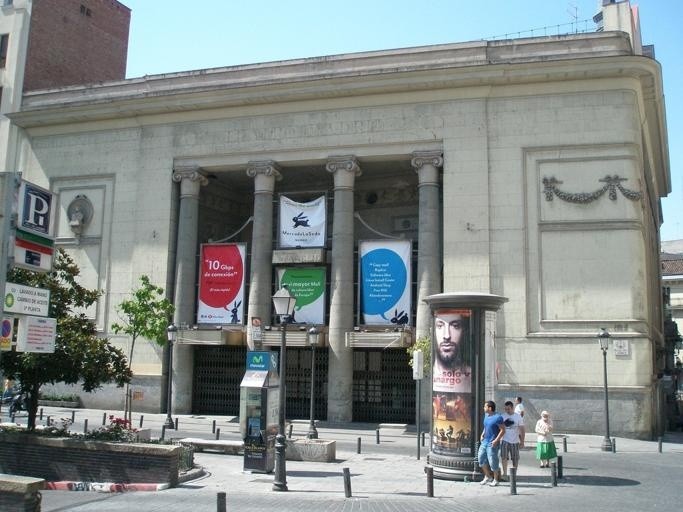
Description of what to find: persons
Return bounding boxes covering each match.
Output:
[445,425,453,443]
[514,396,525,448]
[434,311,471,393]
[477,401,506,486]
[500,401,526,477]
[2,379,13,405]
[534,410,558,468]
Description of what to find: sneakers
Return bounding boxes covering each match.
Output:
[480,475,499,487]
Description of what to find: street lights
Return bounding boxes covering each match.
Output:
[271,282,295,492]
[596,325,613,452]
[163,321,177,428]
[307,322,319,439]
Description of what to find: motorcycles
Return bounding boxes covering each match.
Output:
[8,388,43,416]
